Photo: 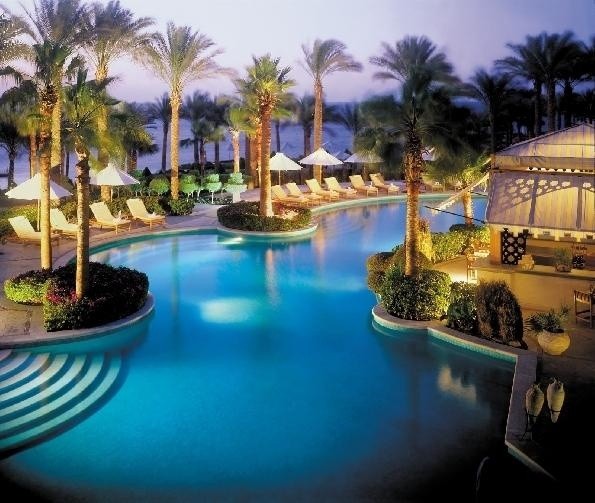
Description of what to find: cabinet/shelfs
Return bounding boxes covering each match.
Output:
[573,286,595,329]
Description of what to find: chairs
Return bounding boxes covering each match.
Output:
[271,170,465,208]
[6,196,167,251]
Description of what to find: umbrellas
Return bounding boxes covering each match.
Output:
[298,146,346,185]
[4,172,74,232]
[90,162,140,202]
[255,150,303,186]
[341,147,388,175]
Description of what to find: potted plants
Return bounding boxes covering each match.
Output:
[523,302,574,358]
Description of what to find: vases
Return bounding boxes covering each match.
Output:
[525,374,566,431]
